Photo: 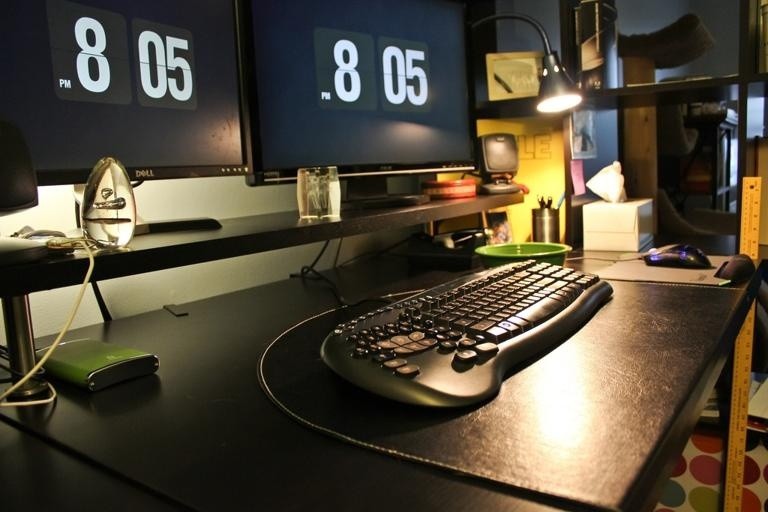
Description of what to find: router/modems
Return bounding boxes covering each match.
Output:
[35,339,158,392]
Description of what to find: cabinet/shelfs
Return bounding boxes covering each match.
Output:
[561,2,768,257]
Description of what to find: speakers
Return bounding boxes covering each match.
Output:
[0,122,48,267]
[475,133,519,194]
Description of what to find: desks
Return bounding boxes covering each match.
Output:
[1,232,766,512]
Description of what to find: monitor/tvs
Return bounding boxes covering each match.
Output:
[242,0,478,212]
[0,0,253,237]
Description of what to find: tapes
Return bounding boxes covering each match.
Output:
[432,236,455,249]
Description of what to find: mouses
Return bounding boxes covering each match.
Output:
[643,244,711,269]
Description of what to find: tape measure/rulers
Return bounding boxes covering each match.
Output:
[723,175,761,512]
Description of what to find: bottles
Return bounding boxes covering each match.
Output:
[674,101,725,118]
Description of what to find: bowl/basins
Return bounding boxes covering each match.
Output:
[476,242,573,270]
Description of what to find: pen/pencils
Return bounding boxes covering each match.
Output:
[537,190,566,210]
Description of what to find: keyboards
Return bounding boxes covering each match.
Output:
[320,258,614,406]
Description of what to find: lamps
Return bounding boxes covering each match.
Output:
[468,14,583,115]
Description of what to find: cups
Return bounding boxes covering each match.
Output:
[296,165,342,220]
[531,208,560,243]
